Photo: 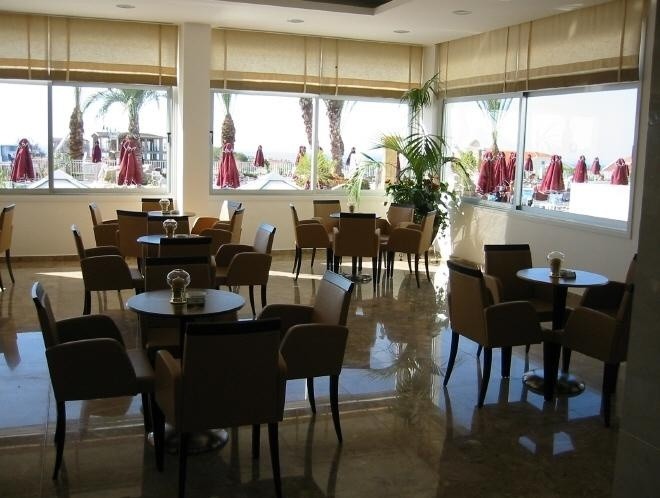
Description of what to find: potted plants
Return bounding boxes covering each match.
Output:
[341,70,471,245]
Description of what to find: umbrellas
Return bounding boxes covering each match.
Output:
[571,155,588,183]
[119,136,143,184]
[254,145,265,166]
[477,150,534,195]
[93,140,102,161]
[11,138,36,182]
[611,159,630,185]
[539,153,566,194]
[217,142,241,188]
[590,156,601,174]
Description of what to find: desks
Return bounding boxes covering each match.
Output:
[514,266,610,398]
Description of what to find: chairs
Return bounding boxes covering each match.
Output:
[551,253,639,429]
[484,244,556,352]
[443,257,544,409]
[0,202,17,293]
